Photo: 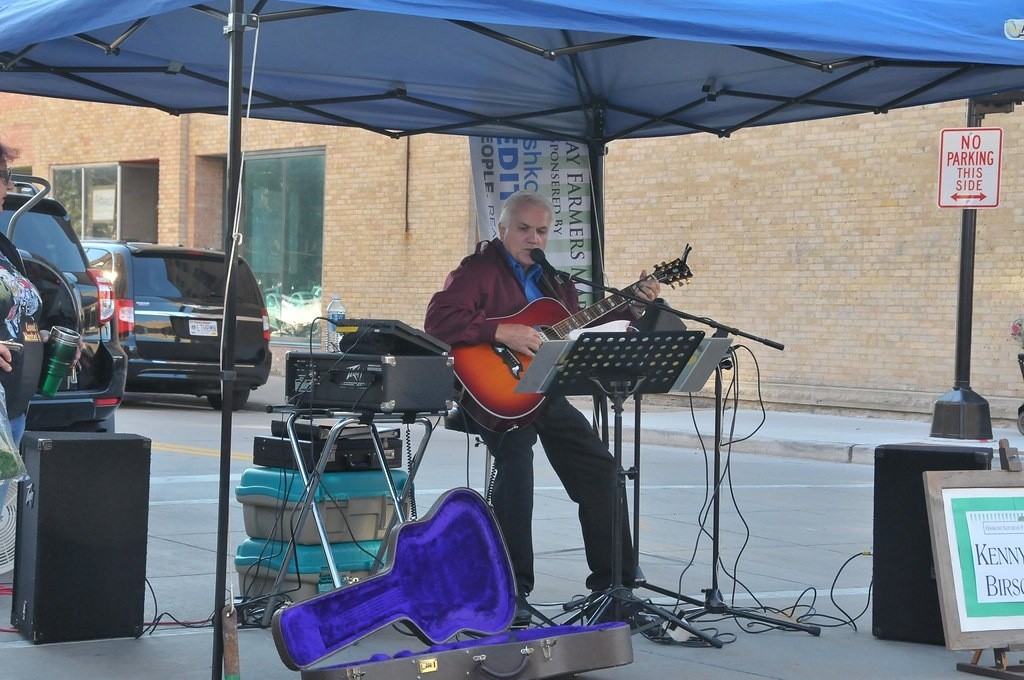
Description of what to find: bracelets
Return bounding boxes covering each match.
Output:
[629,300,645,318]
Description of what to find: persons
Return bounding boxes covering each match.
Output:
[424,191,661,627]
[1,145,85,532]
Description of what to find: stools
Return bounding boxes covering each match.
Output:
[445,406,492,503]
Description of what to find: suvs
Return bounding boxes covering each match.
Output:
[0,181,130,434]
[78,237,271,413]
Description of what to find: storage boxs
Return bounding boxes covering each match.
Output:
[235,470,411,544]
[234,537,388,608]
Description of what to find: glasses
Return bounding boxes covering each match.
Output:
[0,169,13,183]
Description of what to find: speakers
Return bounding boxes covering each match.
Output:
[10,431,150,646]
[872,445,994,648]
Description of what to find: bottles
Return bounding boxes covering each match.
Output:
[326,292,346,352]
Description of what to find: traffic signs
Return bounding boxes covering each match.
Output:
[937,127,1004,209]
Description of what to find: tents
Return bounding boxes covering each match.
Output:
[1,1,1023,680]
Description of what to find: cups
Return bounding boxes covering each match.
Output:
[35,326,80,398]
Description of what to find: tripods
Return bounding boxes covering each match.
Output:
[513,267,821,649]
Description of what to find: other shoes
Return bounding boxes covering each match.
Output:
[589,590,651,620]
[511,598,533,627]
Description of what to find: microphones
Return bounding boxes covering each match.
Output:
[530,247,564,285]
